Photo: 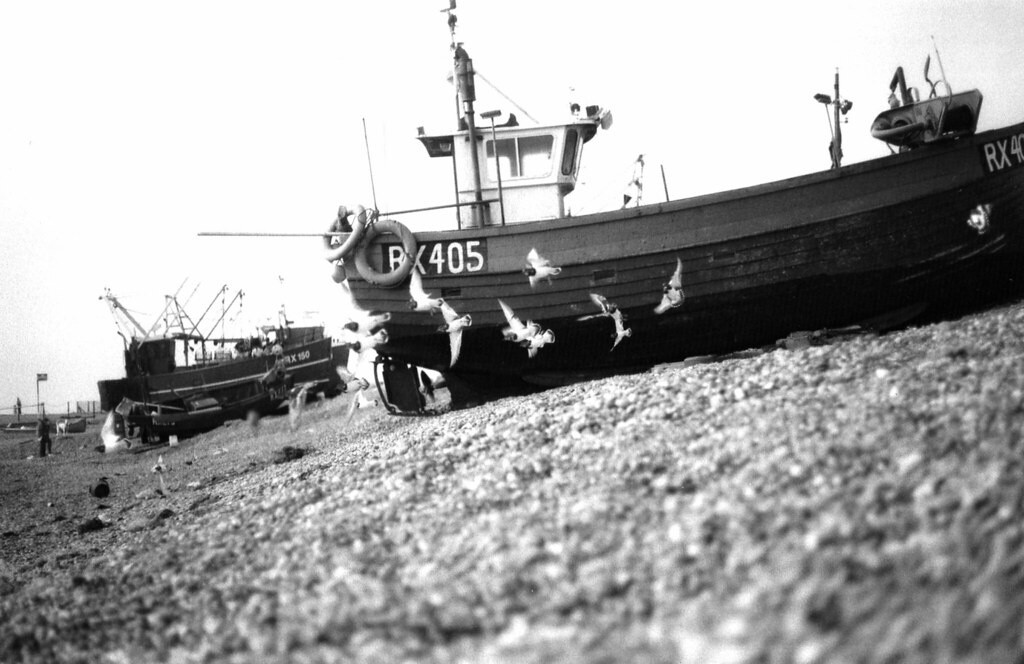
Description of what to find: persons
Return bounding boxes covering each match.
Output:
[36,416,52,457]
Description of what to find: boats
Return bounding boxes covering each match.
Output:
[869,88,982,150]
[57,416,87,433]
[197,0,1024,409]
[96,274,360,444]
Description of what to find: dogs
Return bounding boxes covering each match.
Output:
[56,418,71,436]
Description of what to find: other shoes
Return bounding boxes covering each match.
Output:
[49,454,55,456]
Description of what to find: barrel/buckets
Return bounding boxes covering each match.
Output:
[89,480,109,497]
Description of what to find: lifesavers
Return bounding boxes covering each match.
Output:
[320,204,367,261]
[354,219,418,286]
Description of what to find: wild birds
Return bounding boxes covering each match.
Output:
[94,408,133,453]
[967,203,994,235]
[275,312,393,430]
[409,247,687,368]
